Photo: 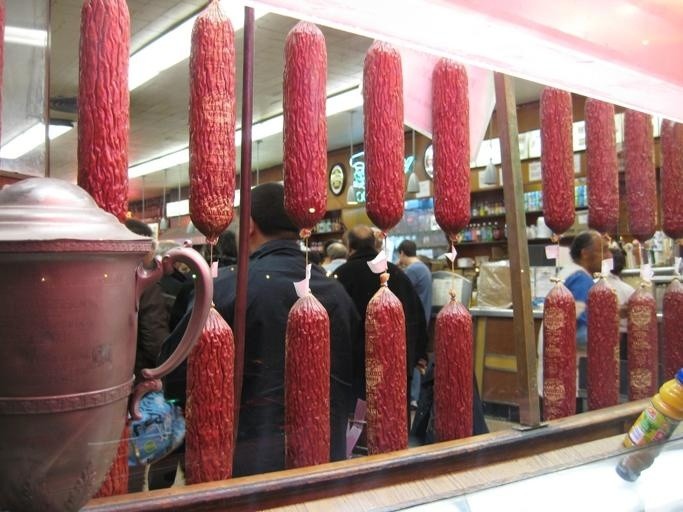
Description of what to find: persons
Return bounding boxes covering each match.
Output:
[395,239,433,410]
[594,247,636,360]
[170,229,237,335]
[326,242,348,277]
[155,182,366,480]
[124,218,170,492]
[147,241,187,490]
[536,229,614,423]
[328,223,428,435]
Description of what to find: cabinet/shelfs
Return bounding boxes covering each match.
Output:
[386,179,681,316]
[119,208,347,271]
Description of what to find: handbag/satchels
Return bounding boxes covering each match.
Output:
[125,391,185,470]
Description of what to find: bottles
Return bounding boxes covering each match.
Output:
[615,369,683,451]
[473,199,503,216]
[460,220,501,241]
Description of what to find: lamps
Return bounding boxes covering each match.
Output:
[346,185,358,203]
[482,159,499,186]
[1,121,74,162]
[184,219,196,236]
[2,20,50,48]
[128,1,269,102]
[406,173,420,194]
[158,215,170,231]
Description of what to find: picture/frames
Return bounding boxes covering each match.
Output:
[327,163,346,197]
